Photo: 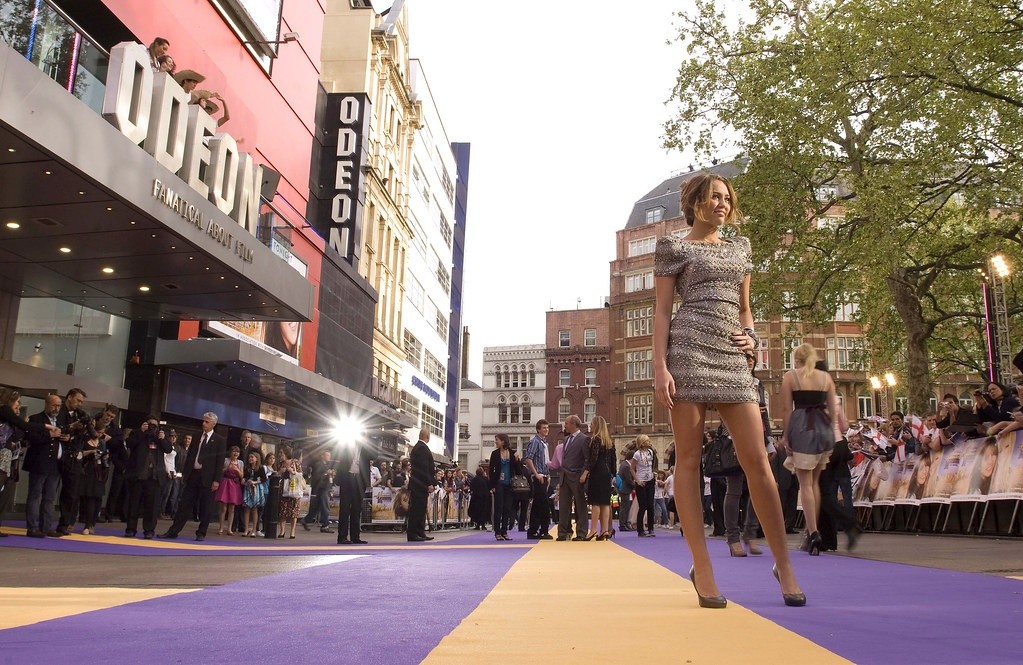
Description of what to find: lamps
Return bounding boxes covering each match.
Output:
[245,32,299,43]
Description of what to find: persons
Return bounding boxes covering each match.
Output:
[215,430,335,539]
[848,349,1023,466]
[905,451,932,499]
[147,36,230,181]
[0,386,61,539]
[160,427,193,520]
[20,388,172,540]
[663,349,763,556]
[515,415,616,541]
[763,343,858,556]
[333,424,523,544]
[966,436,998,495]
[860,468,881,502]
[156,412,226,541]
[654,173,807,609]
[619,434,675,538]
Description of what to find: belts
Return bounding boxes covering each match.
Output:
[347,473,361,476]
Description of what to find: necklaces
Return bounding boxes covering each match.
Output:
[639,449,649,466]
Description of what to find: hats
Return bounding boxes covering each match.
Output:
[167,428,178,436]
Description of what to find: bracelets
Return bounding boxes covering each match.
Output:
[743,327,761,350]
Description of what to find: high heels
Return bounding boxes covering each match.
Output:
[501,534,512,540]
[772,564,806,606]
[808,529,822,557]
[744,539,764,554]
[689,567,727,609]
[494,534,505,541]
[728,543,748,557]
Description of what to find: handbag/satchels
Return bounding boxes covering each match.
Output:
[703,437,743,477]
[282,470,303,498]
[511,475,530,493]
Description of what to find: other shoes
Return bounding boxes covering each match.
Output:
[124,531,136,537]
[525,524,658,542]
[350,538,368,546]
[24,523,97,537]
[301,519,309,530]
[195,534,206,541]
[419,534,434,541]
[338,538,353,546]
[215,526,296,540]
[407,535,426,542]
[157,531,178,540]
[319,527,335,534]
[143,531,153,540]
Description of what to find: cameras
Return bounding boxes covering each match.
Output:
[973,390,989,396]
[941,402,949,407]
[147,423,157,430]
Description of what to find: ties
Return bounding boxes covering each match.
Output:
[566,434,574,449]
[197,434,209,465]
